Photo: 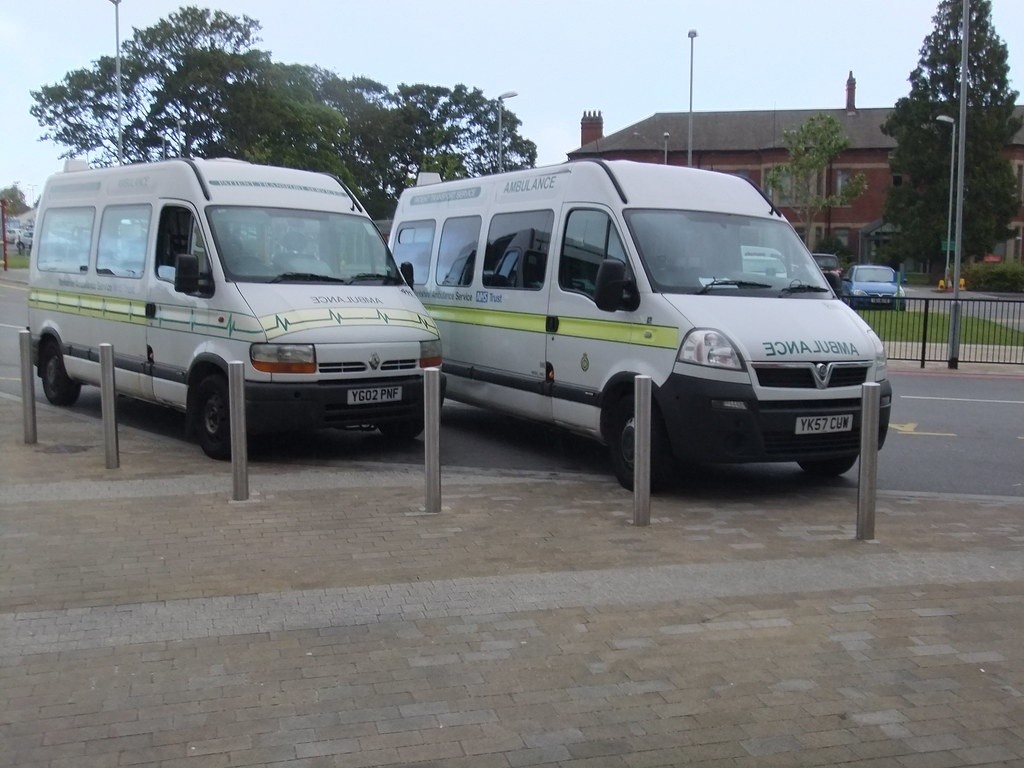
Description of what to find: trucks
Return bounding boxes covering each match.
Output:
[738,246,788,282]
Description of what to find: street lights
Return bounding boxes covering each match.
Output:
[936,115,956,290]
[687,29,697,166]
[498,90,519,172]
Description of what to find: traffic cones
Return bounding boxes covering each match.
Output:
[937,279,946,291]
[959,278,966,291]
[946,277,953,293]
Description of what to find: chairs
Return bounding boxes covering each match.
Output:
[282,231,333,275]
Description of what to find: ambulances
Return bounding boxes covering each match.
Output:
[382,154,894,497]
[26,156,447,456]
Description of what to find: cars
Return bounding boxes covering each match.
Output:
[839,263,906,311]
[4,218,34,252]
[807,252,843,293]
